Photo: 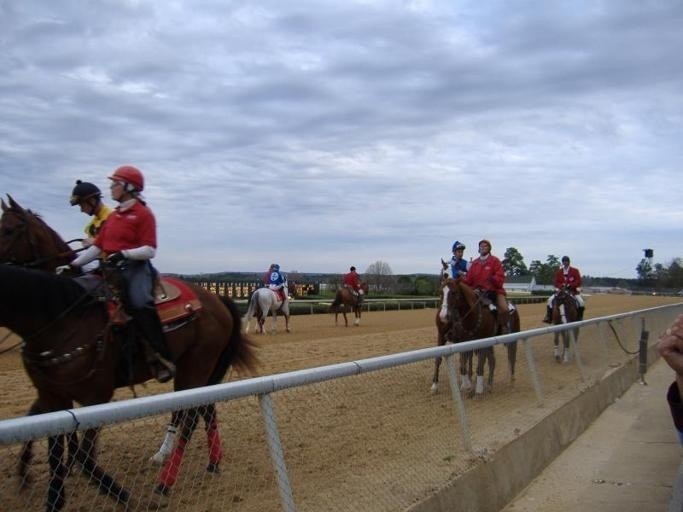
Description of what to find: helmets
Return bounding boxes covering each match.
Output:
[452,240,466,252]
[478,240,492,248]
[108,166,144,189]
[69,180,100,205]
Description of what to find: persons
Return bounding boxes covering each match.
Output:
[343,266,361,299]
[656,313,683,443]
[269,264,287,300]
[70,179,113,263]
[447,241,469,275]
[459,240,507,314]
[55,165,173,383]
[263,264,274,288]
[542,256,585,323]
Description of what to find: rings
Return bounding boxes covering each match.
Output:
[666,329,672,336]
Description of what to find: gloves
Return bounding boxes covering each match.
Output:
[108,253,124,266]
[56,265,69,275]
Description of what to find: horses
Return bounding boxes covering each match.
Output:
[0,194,241,493]
[330,280,369,328]
[553,283,577,362]
[241,273,291,335]
[430,277,520,395]
[439,258,495,390]
[0,264,267,512]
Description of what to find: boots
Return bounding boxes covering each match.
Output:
[144,346,173,384]
[498,325,509,336]
[543,307,553,324]
